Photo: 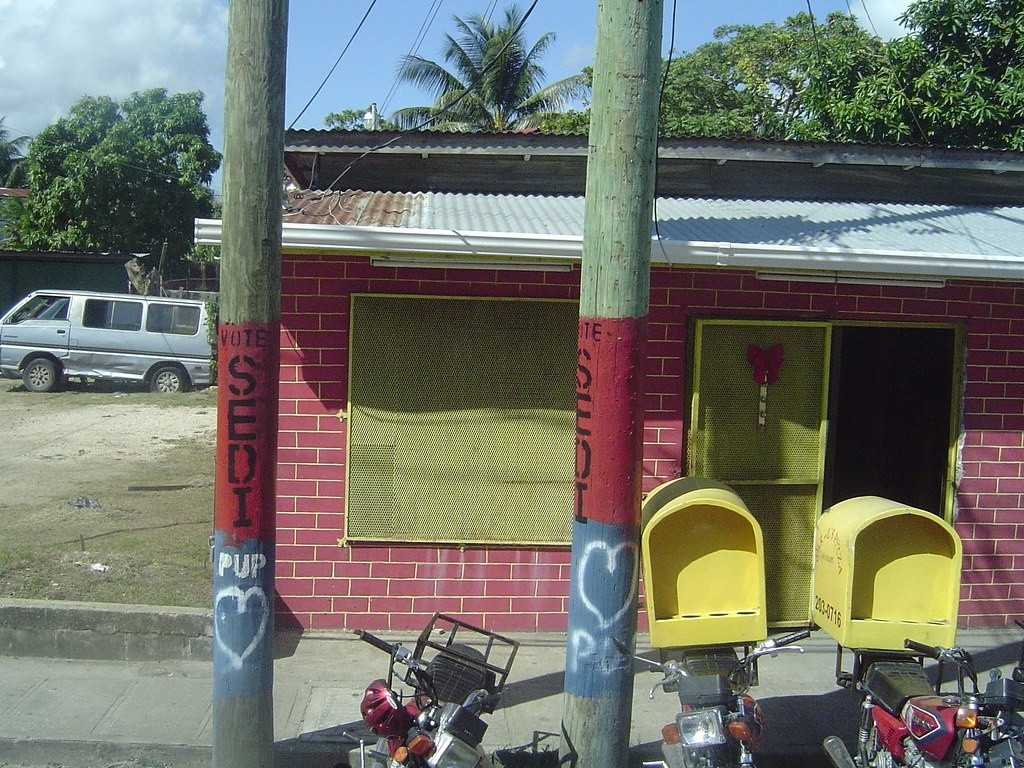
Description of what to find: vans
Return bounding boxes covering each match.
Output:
[0,289,216,392]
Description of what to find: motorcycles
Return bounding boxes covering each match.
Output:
[823,644,1024,768]
[343,610,520,768]
[612,630,811,768]
